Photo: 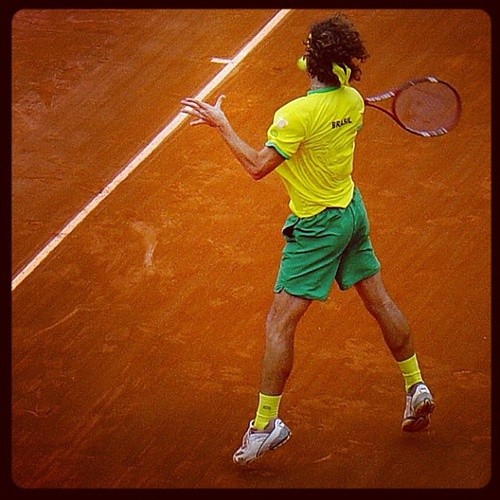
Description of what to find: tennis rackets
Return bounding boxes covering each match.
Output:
[364,76,462,137]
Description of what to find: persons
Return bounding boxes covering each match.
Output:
[180,17,435,466]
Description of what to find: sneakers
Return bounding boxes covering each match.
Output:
[232,419,292,465]
[401,385,435,433]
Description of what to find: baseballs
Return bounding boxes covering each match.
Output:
[296,56,307,71]
